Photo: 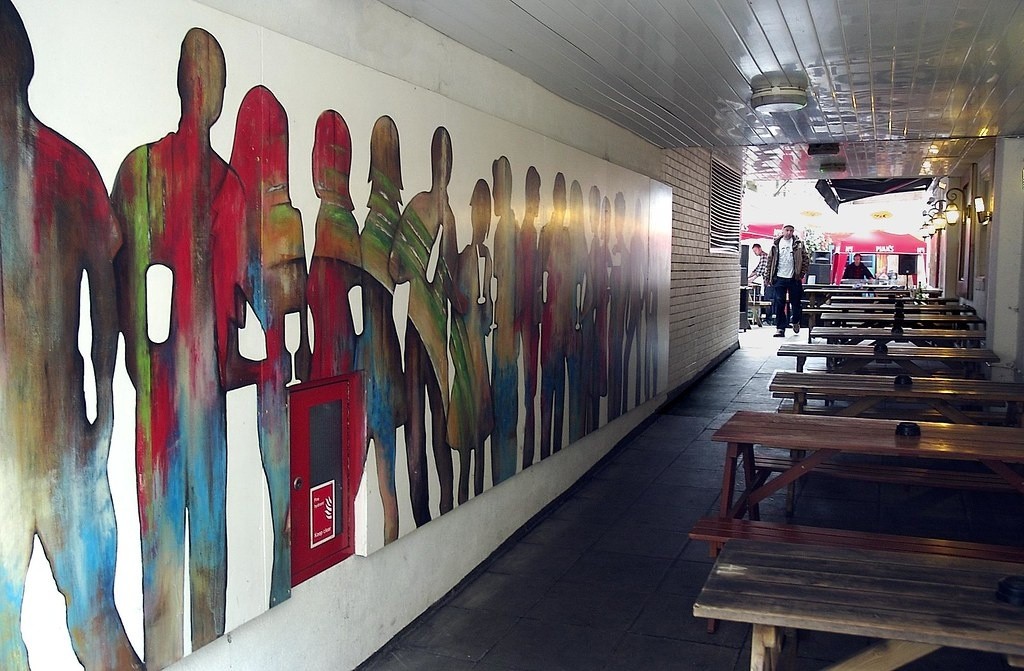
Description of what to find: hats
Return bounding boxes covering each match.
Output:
[783,222,795,228]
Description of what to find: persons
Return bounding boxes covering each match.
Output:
[839,253,874,304]
[763,224,810,337]
[748,243,778,327]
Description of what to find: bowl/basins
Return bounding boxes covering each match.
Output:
[891,327,903,333]
[874,343,888,351]
[895,422,921,435]
[895,301,905,306]
[995,572,1023,606]
[894,374,913,384]
[894,313,905,318]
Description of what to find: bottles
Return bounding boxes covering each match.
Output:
[909,281,929,300]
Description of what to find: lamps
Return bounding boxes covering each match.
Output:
[749,87,807,115]
[919,187,992,241]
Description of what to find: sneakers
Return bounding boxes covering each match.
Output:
[774,329,784,338]
[792,323,800,334]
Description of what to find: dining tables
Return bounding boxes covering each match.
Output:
[687,278,1024,671]
